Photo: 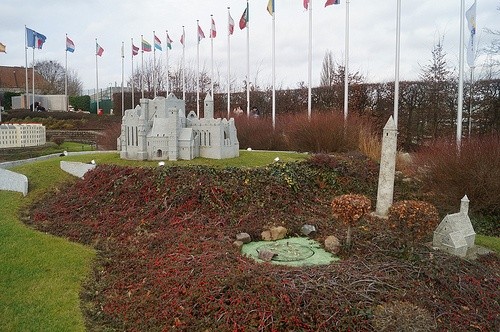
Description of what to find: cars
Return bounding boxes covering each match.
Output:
[68,105,75,112]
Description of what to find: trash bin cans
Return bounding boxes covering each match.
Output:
[99,109,103,114]
[110,108,113,114]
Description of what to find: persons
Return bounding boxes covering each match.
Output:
[30,102,45,112]
[98,108,103,116]
[252,107,261,115]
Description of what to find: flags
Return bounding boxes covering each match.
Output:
[239,8,248,30]
[465,0,478,69]
[26,28,46,49]
[303,0,309,10]
[66,37,75,52]
[180,34,183,45]
[324,0,340,8]
[167,35,173,50]
[133,45,139,56]
[197,25,205,41]
[266,0,274,17]
[229,16,234,35]
[97,43,104,56]
[143,39,152,52]
[154,35,162,51]
[0,42,7,54]
[209,17,216,39]
